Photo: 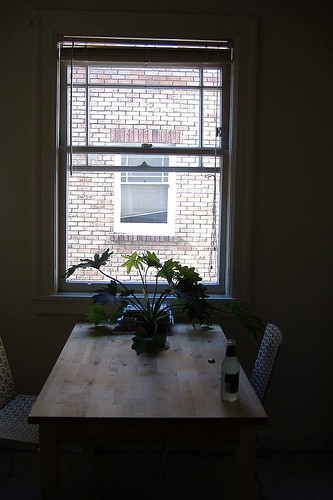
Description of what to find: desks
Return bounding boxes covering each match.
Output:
[26,322,268,499]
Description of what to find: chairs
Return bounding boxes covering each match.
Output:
[153,321,282,499]
[0,336,97,499]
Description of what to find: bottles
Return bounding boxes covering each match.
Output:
[220,340,240,402]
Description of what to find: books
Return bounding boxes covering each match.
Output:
[113,303,175,336]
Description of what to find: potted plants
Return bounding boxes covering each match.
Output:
[60,249,268,357]
